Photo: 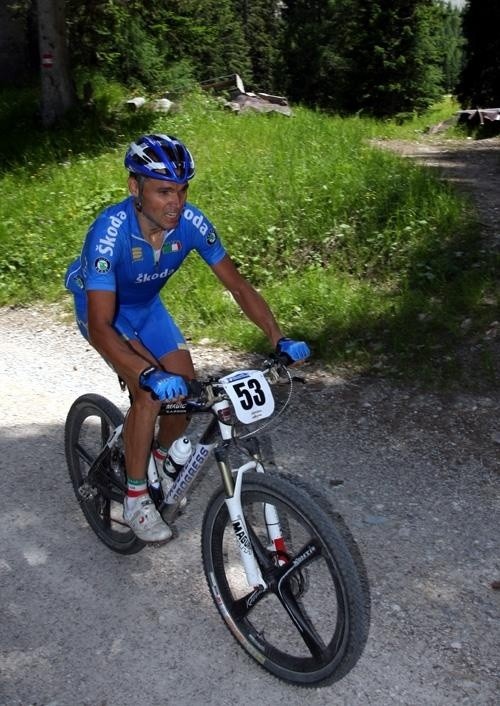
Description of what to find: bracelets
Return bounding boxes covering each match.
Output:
[136,362,158,393]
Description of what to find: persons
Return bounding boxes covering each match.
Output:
[65,133,313,546]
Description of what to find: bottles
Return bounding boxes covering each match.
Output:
[160,436,193,482]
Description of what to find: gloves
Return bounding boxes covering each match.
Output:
[277,336,311,362]
[139,366,188,401]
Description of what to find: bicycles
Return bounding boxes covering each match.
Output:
[64,350,371,688]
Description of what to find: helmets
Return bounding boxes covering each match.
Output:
[124,134,195,184]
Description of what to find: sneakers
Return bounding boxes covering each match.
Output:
[122,495,172,542]
[154,447,188,505]
